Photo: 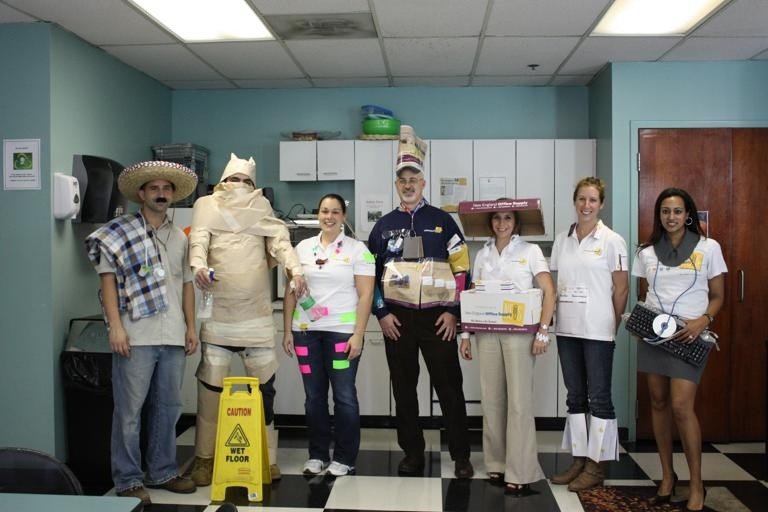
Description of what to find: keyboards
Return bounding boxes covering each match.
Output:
[625,301,716,367]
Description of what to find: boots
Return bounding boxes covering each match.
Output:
[550,457,604,491]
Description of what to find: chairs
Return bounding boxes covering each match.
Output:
[0,446,85,492]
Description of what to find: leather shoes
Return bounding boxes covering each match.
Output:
[399,454,425,473]
[455,459,474,478]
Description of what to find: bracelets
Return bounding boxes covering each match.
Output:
[534,332,550,343]
[539,323,550,330]
[703,313,714,325]
[460,332,471,339]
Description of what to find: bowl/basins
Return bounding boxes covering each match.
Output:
[359,118,400,136]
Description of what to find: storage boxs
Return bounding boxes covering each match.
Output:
[458,287,546,335]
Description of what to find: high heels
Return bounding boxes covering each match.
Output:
[655,472,678,505]
[685,488,707,511]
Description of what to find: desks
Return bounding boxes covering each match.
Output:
[0,487,144,512]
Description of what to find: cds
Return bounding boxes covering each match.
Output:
[653,314,676,339]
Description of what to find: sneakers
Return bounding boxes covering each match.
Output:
[145,477,196,493]
[270,464,280,480]
[303,459,327,474]
[325,461,355,477]
[191,457,214,485]
[116,488,150,506]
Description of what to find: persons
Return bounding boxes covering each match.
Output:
[550,177,630,492]
[188,154,310,487]
[371,124,474,479]
[460,197,556,496]
[85,161,197,503]
[624,187,729,511]
[282,194,376,478]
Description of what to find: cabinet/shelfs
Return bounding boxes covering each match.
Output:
[268,311,630,417]
[354,139,430,242]
[516,138,597,242]
[279,139,354,181]
[152,143,212,207]
[430,139,516,242]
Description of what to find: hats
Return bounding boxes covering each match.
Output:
[118,160,199,203]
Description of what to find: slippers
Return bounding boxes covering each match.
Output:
[490,473,504,481]
[505,483,528,494]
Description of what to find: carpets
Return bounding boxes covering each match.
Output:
[575,486,751,512]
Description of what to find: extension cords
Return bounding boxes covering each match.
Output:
[297,213,318,220]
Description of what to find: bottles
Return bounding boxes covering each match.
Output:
[289,278,326,323]
[197,269,217,320]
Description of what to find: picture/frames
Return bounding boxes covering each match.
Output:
[3,138,41,191]
